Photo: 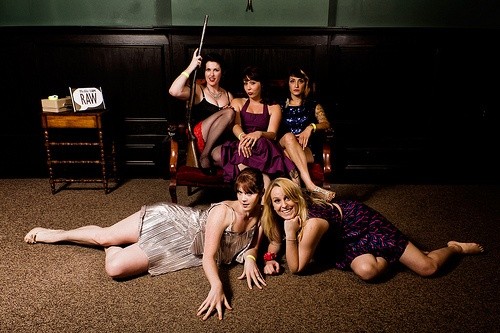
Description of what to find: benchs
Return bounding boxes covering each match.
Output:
[167,124,334,205]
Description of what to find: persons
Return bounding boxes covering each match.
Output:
[169,48,336,202]
[24,167,267,321]
[260,178,485,280]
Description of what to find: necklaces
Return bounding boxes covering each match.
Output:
[206,86,223,99]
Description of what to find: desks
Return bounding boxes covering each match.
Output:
[42,110,109,194]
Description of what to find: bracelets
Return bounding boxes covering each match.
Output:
[181,71,190,79]
[245,254,256,261]
[238,131,244,140]
[286,237,297,242]
[264,252,276,260]
[309,123,317,133]
